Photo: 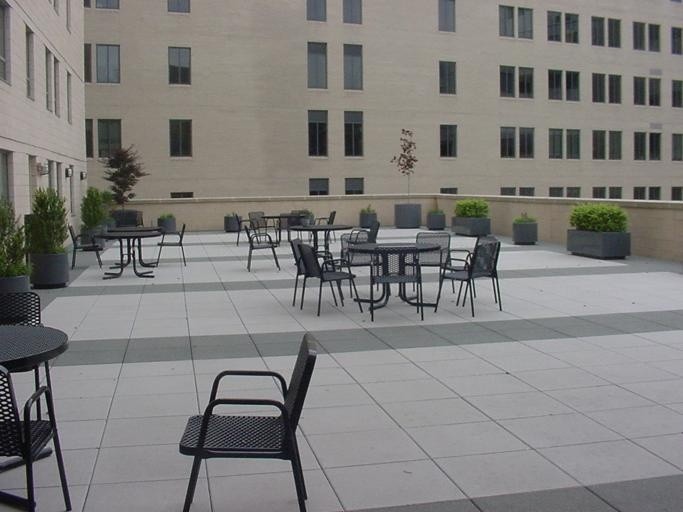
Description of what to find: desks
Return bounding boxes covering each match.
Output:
[94,231,162,279]
[0,325,69,472]
[108,226,157,269]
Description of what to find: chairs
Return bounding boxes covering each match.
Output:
[0,364,72,512]
[156,223,187,267]
[232,211,502,322]
[0,292,42,420]
[178,332,318,512]
[67,225,102,269]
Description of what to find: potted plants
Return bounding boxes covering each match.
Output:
[388,128,423,229]
[80,186,104,251]
[511,215,538,245]
[25,184,70,289]
[157,211,177,234]
[96,142,151,226]
[0,194,32,322]
[224,213,241,233]
[566,202,632,260]
[451,199,492,238]
